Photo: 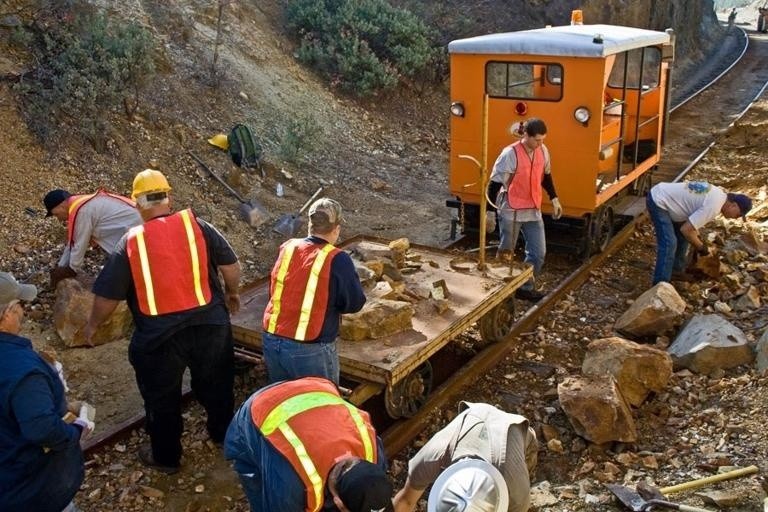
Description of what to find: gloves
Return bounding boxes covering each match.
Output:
[76,401,96,442]
[552,198,564,221]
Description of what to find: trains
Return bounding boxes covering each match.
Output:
[444,6,673,254]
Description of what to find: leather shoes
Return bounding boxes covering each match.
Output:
[136,447,181,475]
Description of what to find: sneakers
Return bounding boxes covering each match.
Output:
[515,285,542,300]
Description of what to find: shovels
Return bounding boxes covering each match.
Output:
[602,465,759,512]
[188,149,273,226]
[272,186,323,239]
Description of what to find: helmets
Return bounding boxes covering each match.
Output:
[428,459,508,511]
[131,169,171,199]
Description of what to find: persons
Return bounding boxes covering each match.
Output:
[45,186,147,271]
[728,7,739,33]
[261,198,367,392]
[486,118,565,303]
[1,268,100,512]
[392,400,541,512]
[645,181,752,286]
[223,374,400,510]
[80,170,240,476]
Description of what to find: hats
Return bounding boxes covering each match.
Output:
[44,189,70,218]
[308,198,349,224]
[336,460,393,512]
[0,273,38,304]
[736,195,751,222]
